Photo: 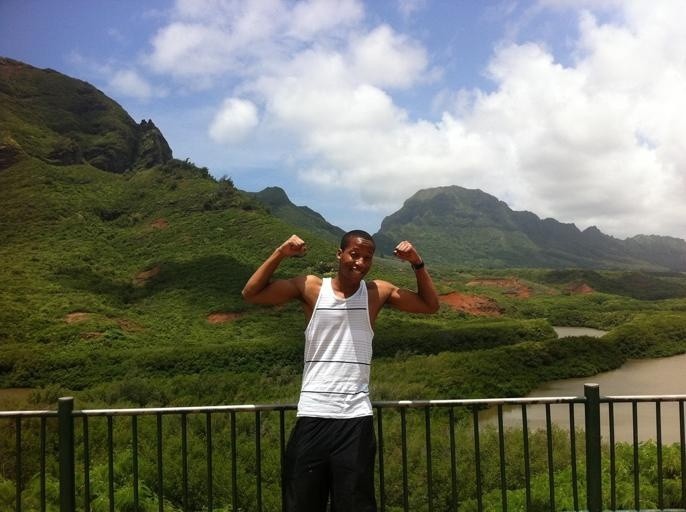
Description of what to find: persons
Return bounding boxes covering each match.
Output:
[243,229,440,512]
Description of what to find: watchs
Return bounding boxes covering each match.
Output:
[411,261,425,272]
